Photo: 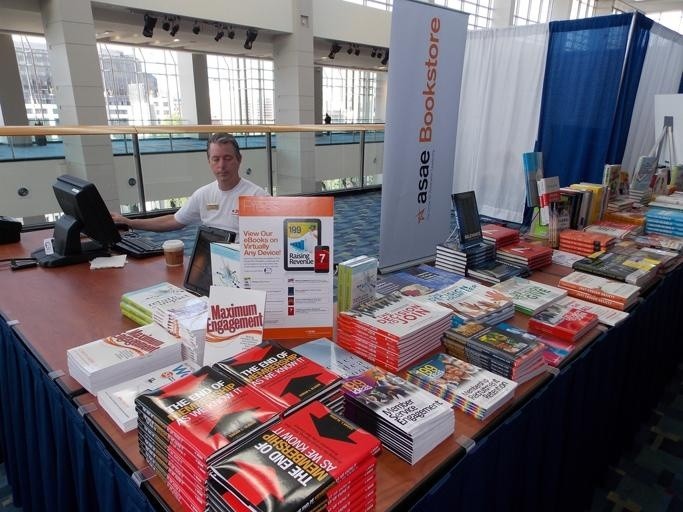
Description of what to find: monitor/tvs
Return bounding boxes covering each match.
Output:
[31,175,123,268]
[184,225,236,298]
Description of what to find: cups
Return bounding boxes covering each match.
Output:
[161,239,185,267]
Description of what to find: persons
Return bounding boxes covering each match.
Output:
[106,128,272,243]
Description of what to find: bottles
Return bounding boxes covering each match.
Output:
[34,121,47,146]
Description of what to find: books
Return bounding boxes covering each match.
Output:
[523,153,683,225]
[428,189,683,384]
[64,246,460,511]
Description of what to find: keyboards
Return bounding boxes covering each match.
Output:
[116,232,164,257]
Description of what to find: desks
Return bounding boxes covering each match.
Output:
[0,214,683,508]
